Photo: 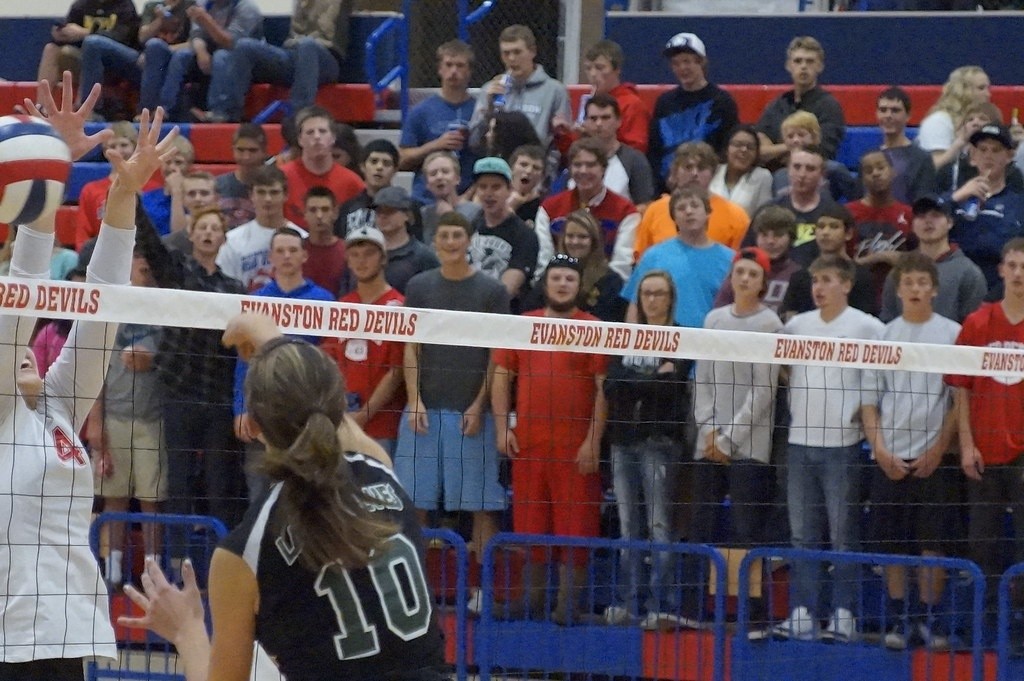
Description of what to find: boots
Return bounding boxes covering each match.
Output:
[493,563,549,621]
[550,564,584,623]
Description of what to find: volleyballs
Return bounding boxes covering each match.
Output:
[0,114,73,226]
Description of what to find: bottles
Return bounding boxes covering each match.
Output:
[965,196,981,221]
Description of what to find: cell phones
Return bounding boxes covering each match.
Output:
[55,21,66,29]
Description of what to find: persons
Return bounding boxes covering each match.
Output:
[38,0,1024,651]
[117,312,446,681]
[0,70,180,681]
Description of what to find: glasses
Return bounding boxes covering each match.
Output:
[549,253,579,268]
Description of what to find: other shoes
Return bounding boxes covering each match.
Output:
[918,620,961,650]
[886,623,913,649]
[85,112,107,123]
[818,625,856,644]
[191,108,228,122]
[771,625,820,640]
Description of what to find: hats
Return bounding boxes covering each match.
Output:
[470,157,512,184]
[969,124,1011,149]
[345,226,386,252]
[912,192,950,217]
[662,33,707,60]
[372,186,409,209]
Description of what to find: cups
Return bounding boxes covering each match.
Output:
[493,74,514,106]
[449,123,467,157]
[579,95,593,124]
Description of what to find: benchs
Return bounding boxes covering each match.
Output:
[0,81,1024,681]
